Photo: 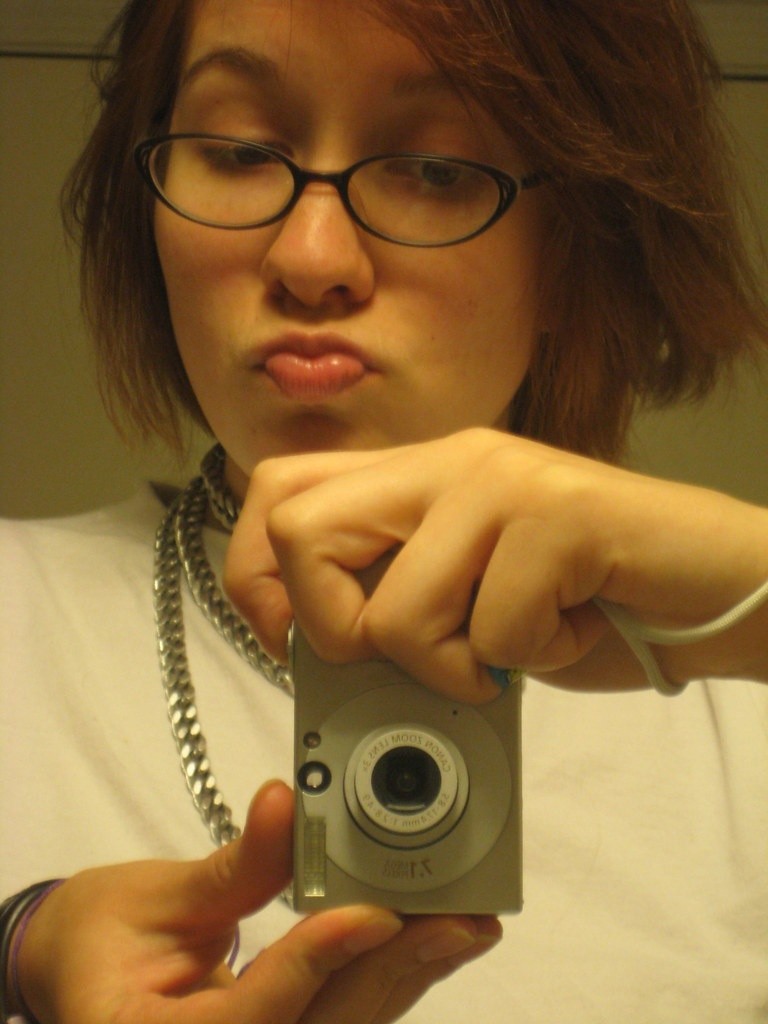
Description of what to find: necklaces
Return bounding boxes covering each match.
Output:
[152,443,299,910]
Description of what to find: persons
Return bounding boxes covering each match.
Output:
[0,0,767,1024]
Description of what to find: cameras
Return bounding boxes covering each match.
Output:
[288,544,524,917]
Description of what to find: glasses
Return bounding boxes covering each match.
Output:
[137,129,561,249]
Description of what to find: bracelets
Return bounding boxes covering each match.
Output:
[0,879,67,1024]
